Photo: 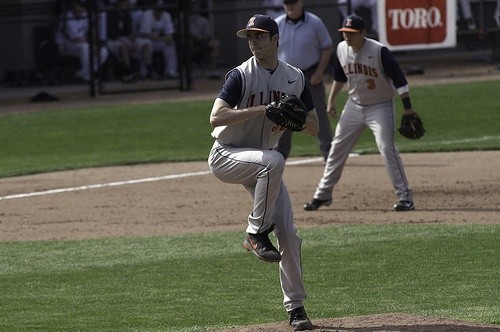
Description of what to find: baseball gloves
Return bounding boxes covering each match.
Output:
[266,94,308,132]
[399,113,426,141]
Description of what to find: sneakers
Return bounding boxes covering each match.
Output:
[242,223,282,262]
[304,197,333,211]
[288,306,312,330]
[394,200,415,212]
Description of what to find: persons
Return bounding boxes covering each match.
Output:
[59,0,476,211]
[209,15,313,332]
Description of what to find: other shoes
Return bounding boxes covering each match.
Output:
[168,71,181,79]
[145,72,155,81]
[122,74,133,82]
[76,71,99,83]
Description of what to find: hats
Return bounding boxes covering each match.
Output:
[283,0,297,4]
[337,13,366,33]
[236,13,279,38]
